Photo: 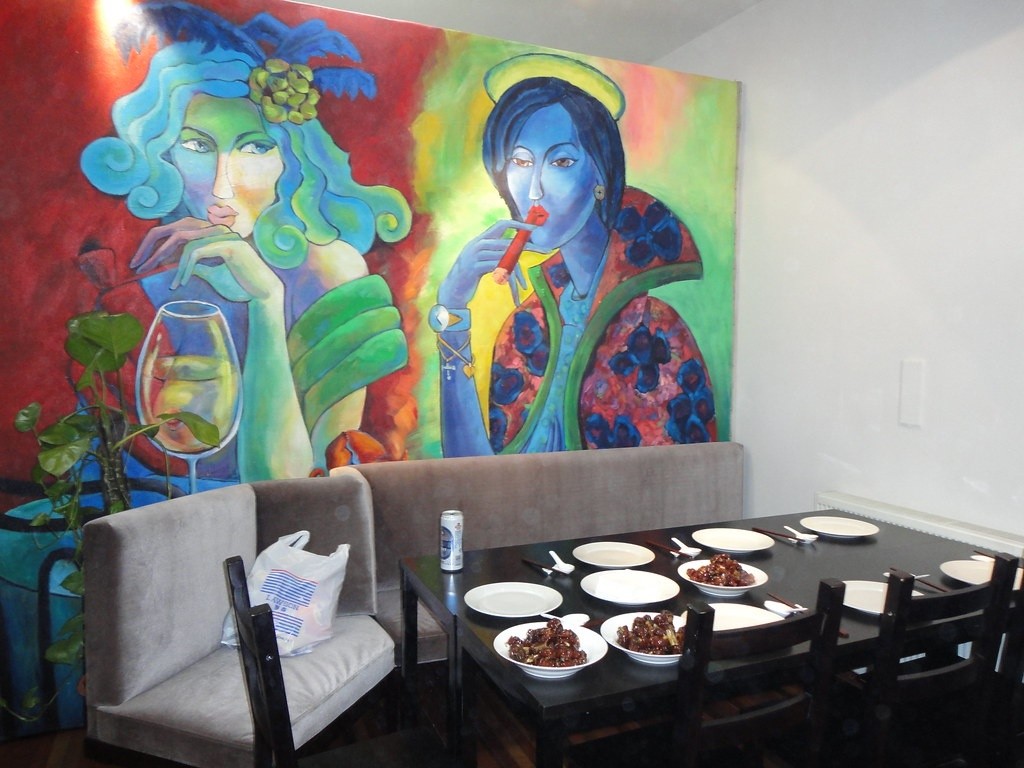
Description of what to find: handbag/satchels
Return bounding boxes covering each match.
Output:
[221,529,352,655]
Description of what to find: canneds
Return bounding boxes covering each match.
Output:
[440,510,464,570]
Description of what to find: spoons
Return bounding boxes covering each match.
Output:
[541,612,590,627]
[549,551,575,574]
[671,537,702,560]
[763,600,809,616]
[783,526,819,544]
[883,572,930,579]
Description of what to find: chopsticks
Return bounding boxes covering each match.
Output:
[973,550,995,558]
[752,527,805,543]
[890,566,946,593]
[522,557,575,580]
[646,541,692,559]
[768,592,849,638]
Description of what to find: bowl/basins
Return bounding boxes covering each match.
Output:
[971,555,995,562]
[493,622,608,678]
[677,560,768,597]
[600,611,685,664]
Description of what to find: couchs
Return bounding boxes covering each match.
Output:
[84,441,759,768]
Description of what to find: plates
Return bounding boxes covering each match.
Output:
[799,516,879,539]
[464,581,563,617]
[842,580,923,616]
[572,542,655,569]
[580,569,681,606]
[938,559,1023,591]
[681,602,784,629]
[692,528,775,555]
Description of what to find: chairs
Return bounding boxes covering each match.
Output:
[865,551,1020,768]
[669,580,844,768]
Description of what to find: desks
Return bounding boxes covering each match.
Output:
[395,512,1024,768]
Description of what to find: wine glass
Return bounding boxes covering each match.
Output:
[135,301,244,495]
